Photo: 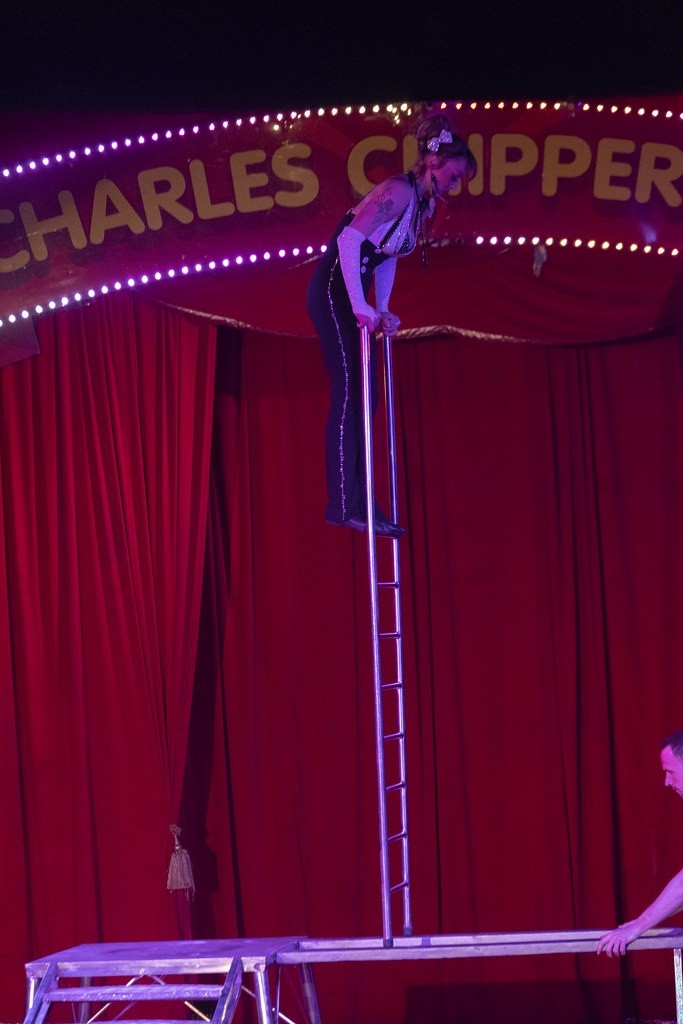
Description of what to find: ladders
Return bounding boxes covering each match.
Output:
[358,320,414,947]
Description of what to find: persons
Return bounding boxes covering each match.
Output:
[305,111,478,539]
[596,731,683,957]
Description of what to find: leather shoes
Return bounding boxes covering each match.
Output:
[326,511,405,538]
